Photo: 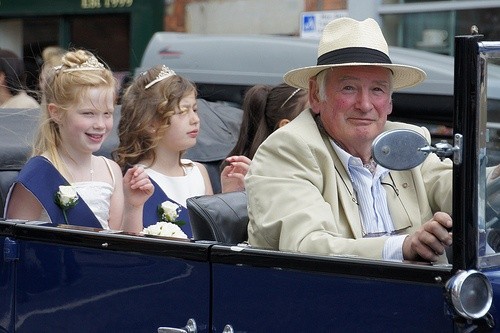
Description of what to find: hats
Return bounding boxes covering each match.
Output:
[282,16,426,91]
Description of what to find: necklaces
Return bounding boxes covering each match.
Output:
[58,148,94,183]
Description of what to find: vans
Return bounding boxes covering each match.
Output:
[131,30,500,127]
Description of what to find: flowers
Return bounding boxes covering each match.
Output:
[157,200,186,226]
[54,184,80,226]
[140,221,188,239]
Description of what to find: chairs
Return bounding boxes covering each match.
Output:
[186,189,251,247]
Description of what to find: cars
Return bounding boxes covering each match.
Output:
[1,25,499,333]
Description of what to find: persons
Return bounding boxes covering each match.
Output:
[245,18,500,261]
[3,42,154,235]
[111,64,252,238]
[0,47,40,108]
[219,80,310,170]
